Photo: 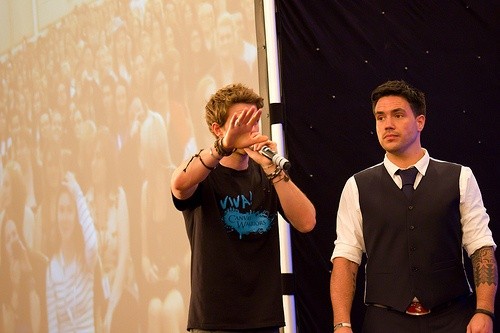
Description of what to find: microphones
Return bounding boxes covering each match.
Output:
[249,144,291,171]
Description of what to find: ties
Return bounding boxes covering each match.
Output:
[399,167,418,199]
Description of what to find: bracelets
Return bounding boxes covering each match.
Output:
[209,145,221,160]
[333,322,351,331]
[182,148,216,173]
[474,308,494,322]
[214,136,237,157]
[263,162,290,184]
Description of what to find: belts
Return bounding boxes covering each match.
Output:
[373,301,431,315]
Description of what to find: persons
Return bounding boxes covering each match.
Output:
[329,80,498,333]
[1,2,258,333]
[170,82,317,333]
